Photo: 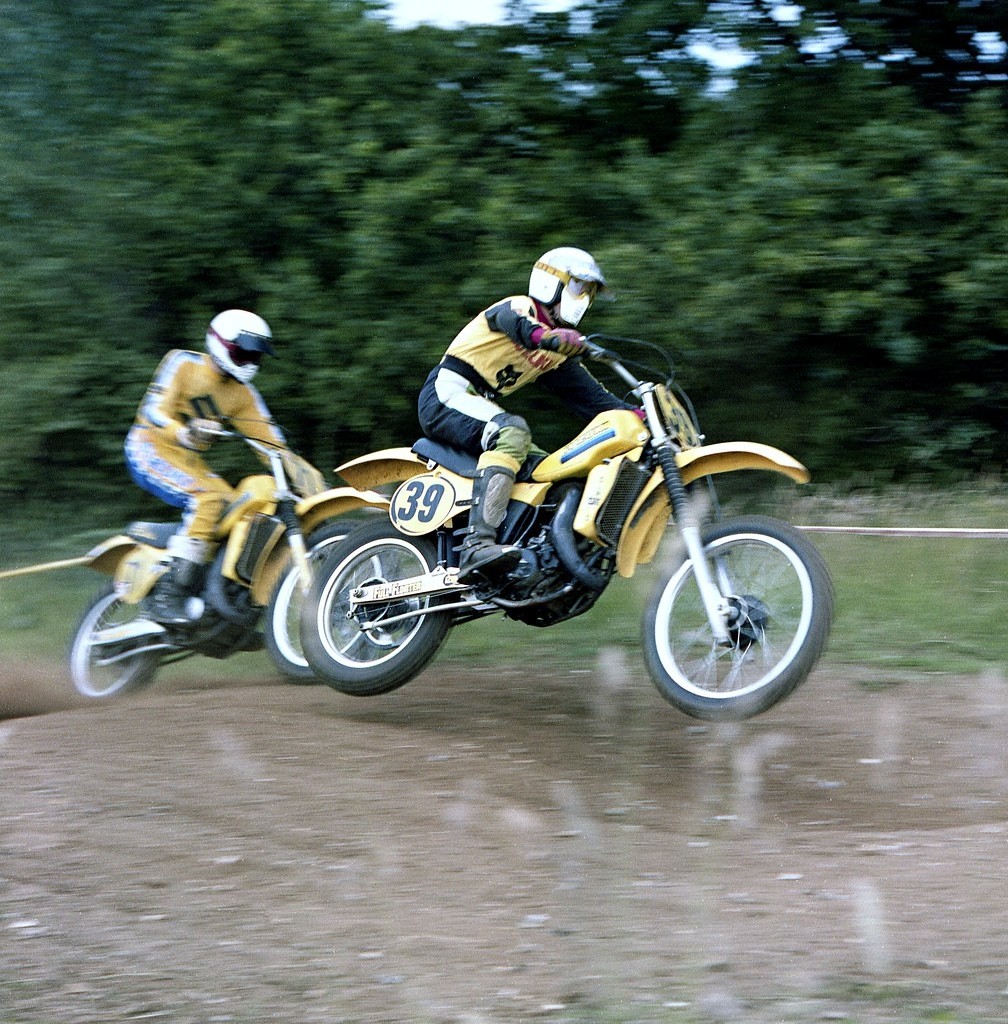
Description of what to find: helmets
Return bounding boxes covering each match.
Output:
[527,246,609,304]
[205,308,281,381]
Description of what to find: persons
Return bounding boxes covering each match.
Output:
[418,246,645,585]
[123,308,322,630]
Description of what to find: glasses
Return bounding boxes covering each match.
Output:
[534,261,599,309]
[209,326,263,368]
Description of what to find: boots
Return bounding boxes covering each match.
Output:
[456,466,522,584]
[136,557,197,631]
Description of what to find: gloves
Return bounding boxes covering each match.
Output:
[190,415,223,442]
[538,329,589,358]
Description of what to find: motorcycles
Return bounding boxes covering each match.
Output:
[64,414,392,708]
[299,331,838,722]
